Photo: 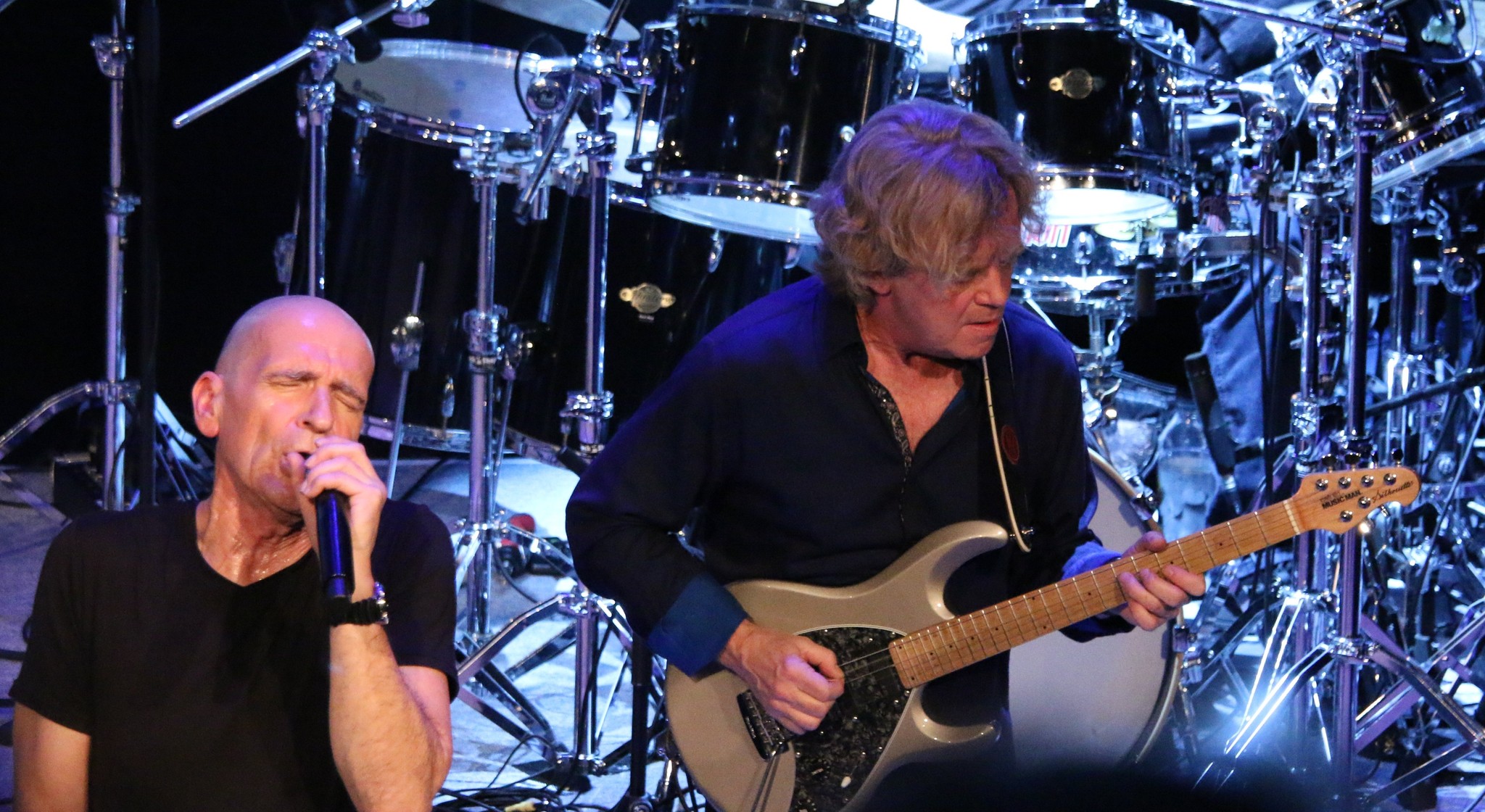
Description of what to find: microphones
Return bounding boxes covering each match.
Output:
[316,487,356,628]
[1183,352,1244,516]
[339,0,383,62]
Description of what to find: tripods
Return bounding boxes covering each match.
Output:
[0,0,217,534]
[1180,0,1485,812]
[448,0,667,779]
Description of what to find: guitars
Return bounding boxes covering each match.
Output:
[663,449,1419,812]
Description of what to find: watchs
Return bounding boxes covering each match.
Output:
[328,579,391,627]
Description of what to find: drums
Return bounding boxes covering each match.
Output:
[959,8,1191,215]
[277,40,630,455]
[640,2,919,243]
[1266,1,1484,195]
[509,171,822,465]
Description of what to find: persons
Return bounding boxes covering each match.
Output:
[7,293,461,812]
[565,98,1205,812]
